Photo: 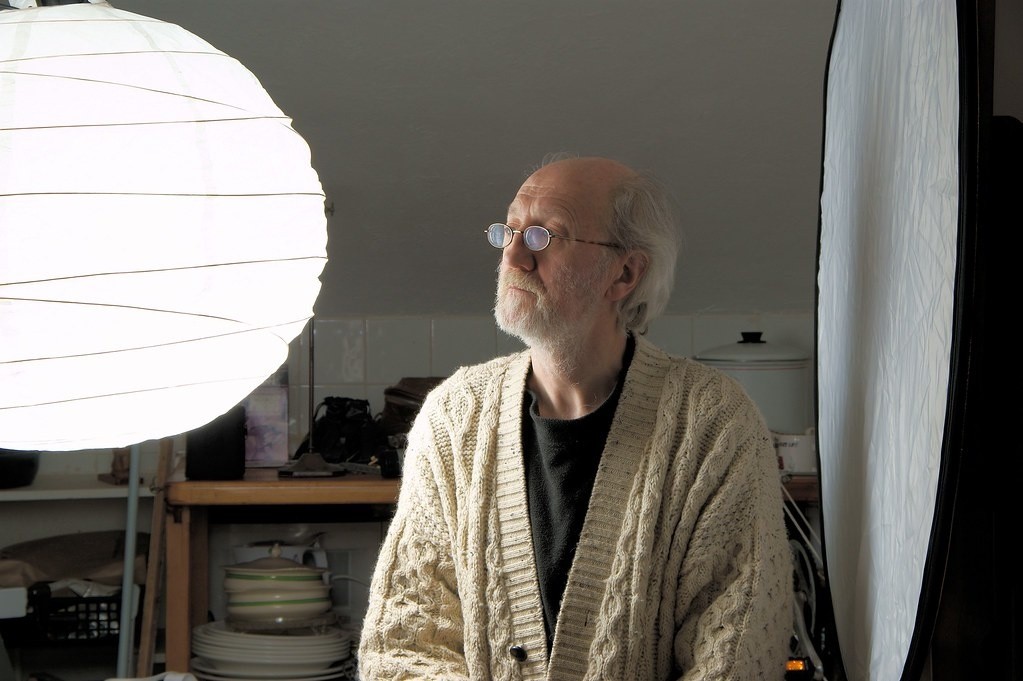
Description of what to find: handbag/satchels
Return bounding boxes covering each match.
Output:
[295,397,373,465]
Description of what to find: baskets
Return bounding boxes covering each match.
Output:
[27,582,121,668]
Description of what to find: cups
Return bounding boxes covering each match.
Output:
[775,434,817,475]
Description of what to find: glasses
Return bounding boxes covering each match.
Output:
[484,223,627,252]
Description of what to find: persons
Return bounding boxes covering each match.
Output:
[358,157,792,681]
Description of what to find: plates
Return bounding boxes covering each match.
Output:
[188,618,355,681]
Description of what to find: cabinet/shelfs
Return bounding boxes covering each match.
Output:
[0,470,830,680]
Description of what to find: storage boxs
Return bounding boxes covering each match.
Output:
[29,578,147,667]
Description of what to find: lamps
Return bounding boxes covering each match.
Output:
[0,4,327,453]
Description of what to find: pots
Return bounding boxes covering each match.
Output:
[219,547,333,623]
